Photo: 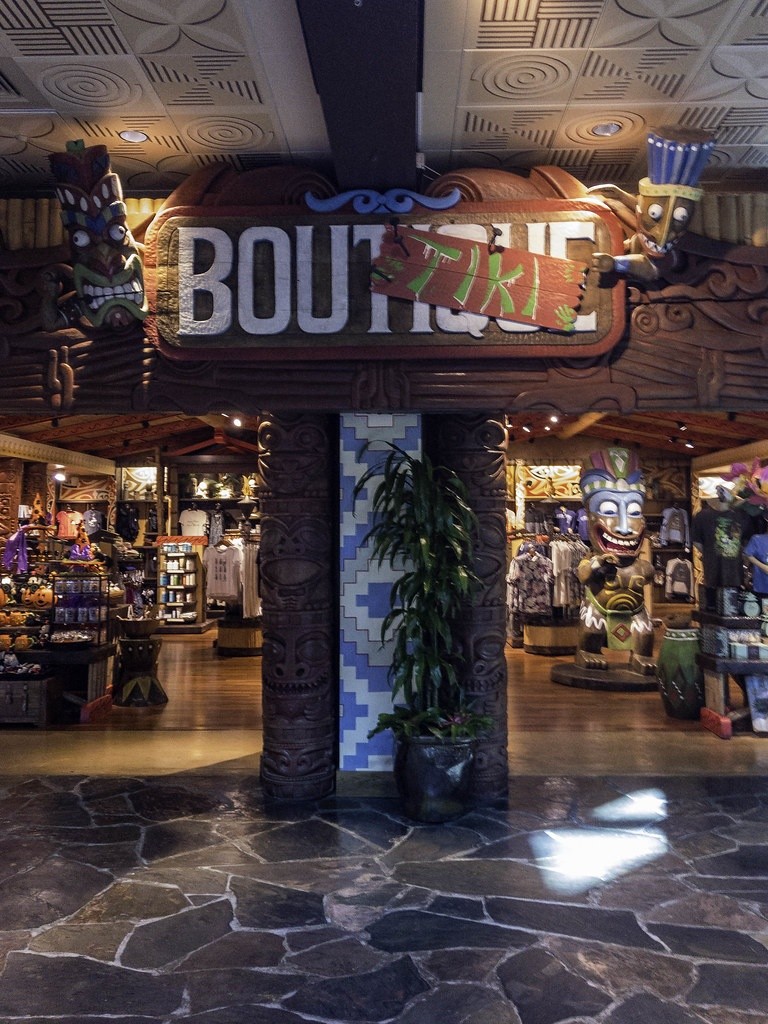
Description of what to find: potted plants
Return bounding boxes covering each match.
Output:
[713,517,741,587]
[350,438,495,820]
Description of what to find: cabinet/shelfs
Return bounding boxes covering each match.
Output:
[691,610,768,737]
[0,533,118,725]
[157,535,208,630]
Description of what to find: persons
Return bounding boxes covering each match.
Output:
[744,530,768,614]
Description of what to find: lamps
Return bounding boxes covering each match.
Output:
[669,436,677,442]
[676,421,687,430]
[522,424,533,433]
[685,439,694,449]
[238,493,261,527]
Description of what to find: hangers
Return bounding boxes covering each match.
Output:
[65,504,74,513]
[523,533,582,556]
[214,532,260,548]
[189,502,198,510]
